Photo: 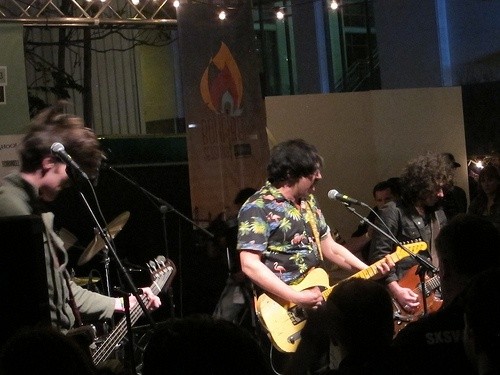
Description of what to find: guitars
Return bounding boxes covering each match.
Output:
[67,254,173,375]
[392,265,444,338]
[255,237,428,354]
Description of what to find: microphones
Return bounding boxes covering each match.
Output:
[328,189,367,207]
[50,142,88,179]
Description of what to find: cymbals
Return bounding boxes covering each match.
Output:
[68,276,101,286]
[77,210,131,265]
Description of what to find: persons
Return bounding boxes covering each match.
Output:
[0,109,162,335]
[0,139,500,375]
